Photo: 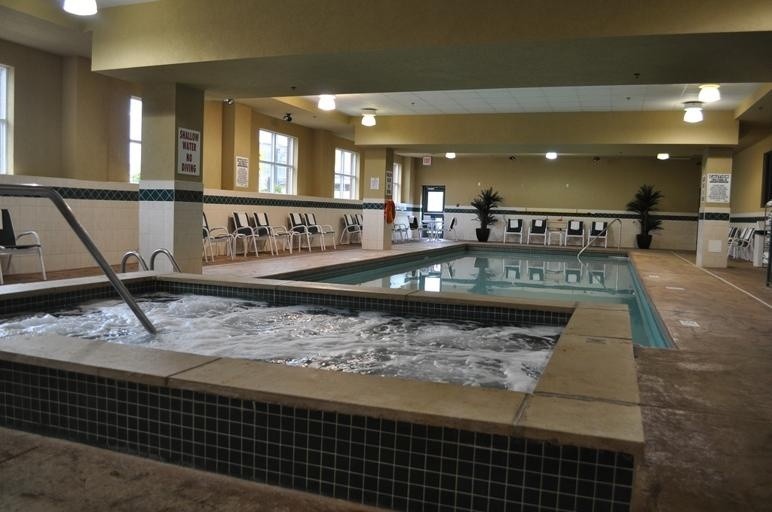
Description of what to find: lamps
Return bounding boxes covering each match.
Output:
[362,109,377,126]
[682,101,703,125]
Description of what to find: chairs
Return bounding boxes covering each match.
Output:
[588,262,606,287]
[0,209,48,285]
[502,256,521,281]
[587,221,609,248]
[202,211,460,266]
[526,259,545,282]
[526,219,547,245]
[563,261,583,283]
[726,226,756,262]
[564,220,585,248]
[504,218,523,244]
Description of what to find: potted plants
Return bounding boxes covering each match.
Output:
[470,187,504,242]
[469,255,498,296]
[626,184,665,248]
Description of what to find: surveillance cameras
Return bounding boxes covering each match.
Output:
[283,116,291,121]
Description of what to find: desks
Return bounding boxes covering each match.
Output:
[544,261,564,273]
[548,227,562,246]
